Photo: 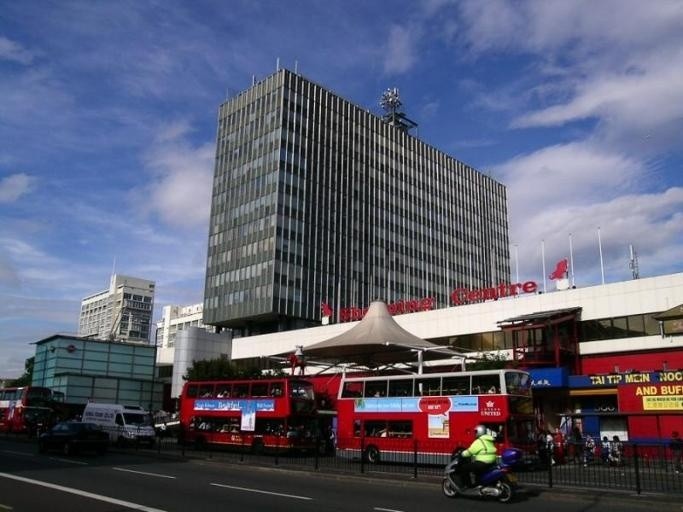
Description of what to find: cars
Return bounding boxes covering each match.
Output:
[38,421,104,449]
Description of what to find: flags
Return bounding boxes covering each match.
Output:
[549,259,567,280]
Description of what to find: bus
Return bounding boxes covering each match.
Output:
[332,369,540,467]
[0,384,52,432]
[177,377,319,454]
[50,390,64,424]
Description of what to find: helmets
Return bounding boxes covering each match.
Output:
[473,425,487,439]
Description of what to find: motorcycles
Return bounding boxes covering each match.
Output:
[441,446,519,502]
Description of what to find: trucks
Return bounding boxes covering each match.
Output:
[79,400,156,443]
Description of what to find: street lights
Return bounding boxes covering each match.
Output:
[149,325,163,411]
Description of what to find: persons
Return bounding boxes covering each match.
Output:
[369,380,499,397]
[372,422,408,438]
[190,417,285,436]
[541,423,627,469]
[454,424,498,493]
[669,430,682,474]
[198,384,283,399]
